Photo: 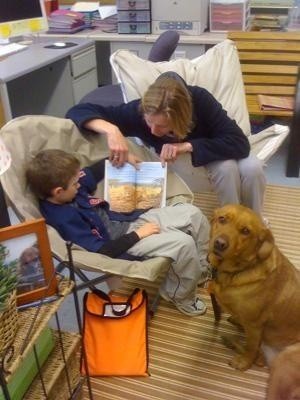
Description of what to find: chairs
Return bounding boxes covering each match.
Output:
[226,32,300,177]
[109,39,290,193]
[0,113,194,324]
[78,30,180,107]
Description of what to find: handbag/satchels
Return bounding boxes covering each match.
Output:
[80,289,147,377]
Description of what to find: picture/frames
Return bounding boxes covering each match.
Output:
[0,218,59,308]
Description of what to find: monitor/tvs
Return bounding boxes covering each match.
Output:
[0,0,49,45]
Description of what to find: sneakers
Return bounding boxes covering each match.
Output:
[157,287,206,316]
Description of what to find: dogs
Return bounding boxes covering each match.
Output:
[205,203,300,400]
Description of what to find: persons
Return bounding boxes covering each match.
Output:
[27,145,213,317]
[113,156,119,158]
[67,71,269,229]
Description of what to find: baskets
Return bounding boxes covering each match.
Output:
[1,289,20,361]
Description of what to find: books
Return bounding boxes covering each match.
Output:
[258,94,293,111]
[102,157,167,214]
[47,8,87,33]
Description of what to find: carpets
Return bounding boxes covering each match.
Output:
[80,184,300,400]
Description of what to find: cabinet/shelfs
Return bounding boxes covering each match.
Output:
[116,0,151,34]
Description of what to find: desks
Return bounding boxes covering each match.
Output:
[24,24,228,86]
[0,37,99,123]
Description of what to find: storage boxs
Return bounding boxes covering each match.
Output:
[209,2,250,32]
